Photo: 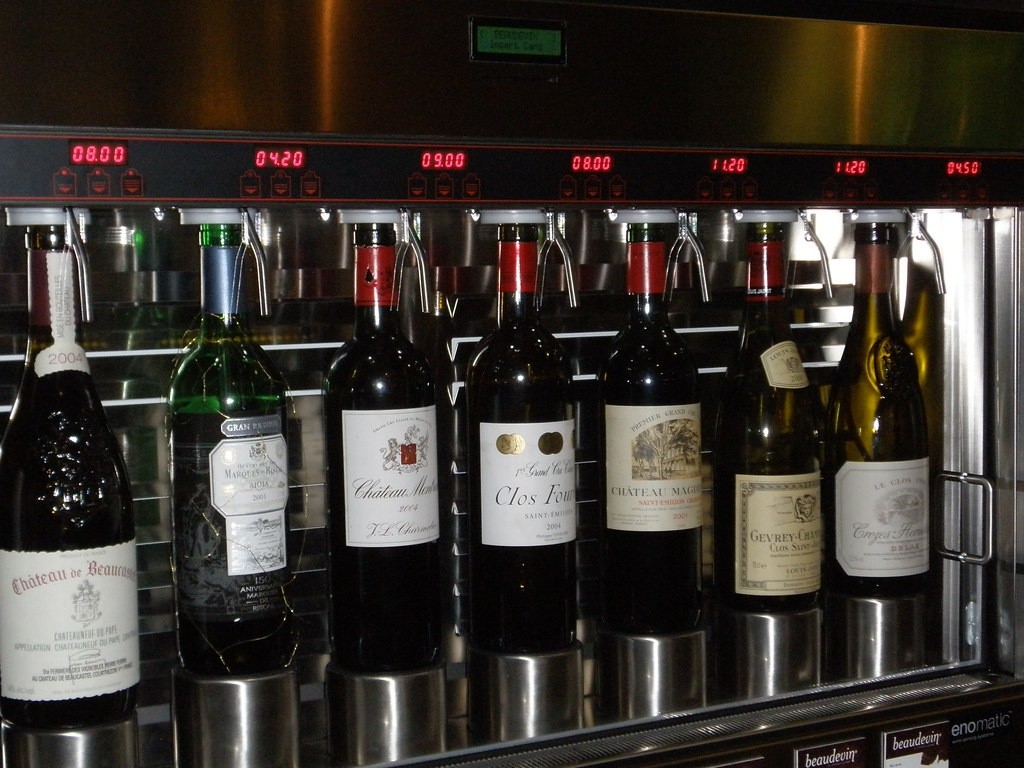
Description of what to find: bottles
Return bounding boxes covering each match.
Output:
[714,221,827,614]
[0,227,141,731]
[163,224,295,677]
[597,223,705,637]
[826,221,934,601]
[463,225,579,652]
[322,225,452,676]
